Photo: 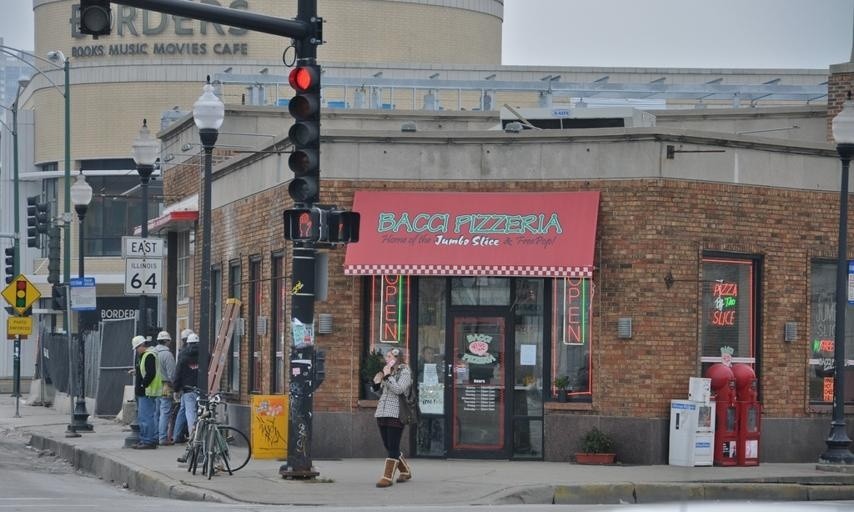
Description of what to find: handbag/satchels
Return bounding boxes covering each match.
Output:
[398,391,421,424]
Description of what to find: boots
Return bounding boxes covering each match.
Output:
[376,458,400,487]
[397,451,413,482]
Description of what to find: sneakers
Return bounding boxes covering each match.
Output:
[132,439,175,449]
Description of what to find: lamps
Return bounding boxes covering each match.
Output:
[151,141,262,172]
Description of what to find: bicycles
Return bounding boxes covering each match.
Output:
[179,381,252,481]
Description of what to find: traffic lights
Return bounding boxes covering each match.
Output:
[4,246,14,285]
[38,201,50,235]
[15,280,27,308]
[285,65,322,205]
[326,208,360,245]
[282,207,321,242]
[49,285,66,311]
[25,195,41,248]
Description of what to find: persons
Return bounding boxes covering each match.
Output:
[416,345,446,383]
[126,335,163,450]
[369,347,415,488]
[153,330,176,445]
[171,329,196,443]
[173,333,210,439]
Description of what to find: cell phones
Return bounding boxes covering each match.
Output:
[388,360,395,366]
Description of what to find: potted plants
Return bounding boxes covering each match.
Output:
[574,426,617,466]
[556,374,570,403]
[363,350,386,401]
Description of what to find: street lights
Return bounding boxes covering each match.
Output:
[69,168,94,432]
[128,114,161,446]
[47,46,70,336]
[819,89,854,466]
[192,73,228,408]
[79,0,111,37]
[9,73,31,398]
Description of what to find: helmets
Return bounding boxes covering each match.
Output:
[181,328,199,343]
[132,335,147,350]
[156,331,172,341]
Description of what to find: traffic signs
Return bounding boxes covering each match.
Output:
[123,257,166,298]
